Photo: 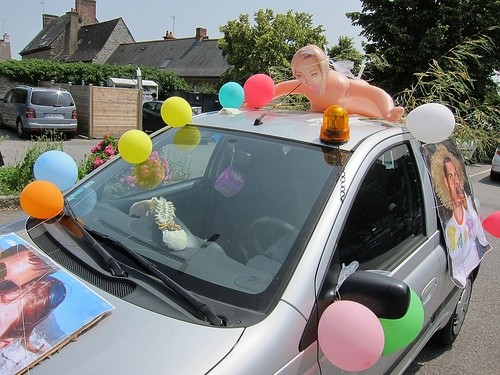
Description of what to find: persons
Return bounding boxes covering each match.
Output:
[275,45,404,121]
[152,93,157,100]
[0,244,67,353]
[442,155,488,287]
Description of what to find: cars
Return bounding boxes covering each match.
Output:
[141,102,211,145]
[490,145,500,181]
[0,107,480,375]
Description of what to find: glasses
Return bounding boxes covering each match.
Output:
[0,262,19,296]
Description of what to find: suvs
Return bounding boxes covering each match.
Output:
[0,84,77,139]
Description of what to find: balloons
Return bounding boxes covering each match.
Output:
[405,102,454,144]
[246,74,274,108]
[118,129,152,165]
[174,127,201,151]
[64,187,96,216]
[218,81,245,111]
[377,287,425,356]
[18,180,64,219]
[318,300,385,372]
[62,217,85,238]
[161,97,192,127]
[481,211,500,239]
[137,160,165,190]
[34,151,78,187]
